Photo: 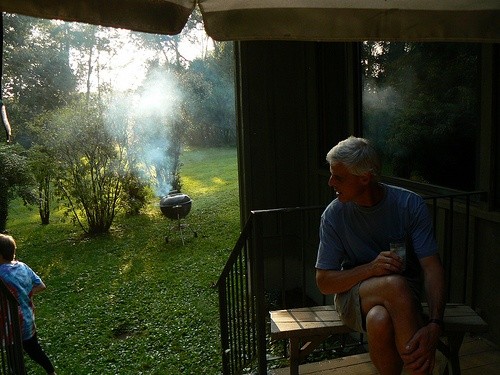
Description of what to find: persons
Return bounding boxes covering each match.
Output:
[1,234,58,374]
[315,135,447,375]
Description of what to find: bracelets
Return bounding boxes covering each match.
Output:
[430,312,446,330]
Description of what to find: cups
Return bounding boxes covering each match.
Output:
[389,241,406,272]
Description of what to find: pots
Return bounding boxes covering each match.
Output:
[160,190,192,220]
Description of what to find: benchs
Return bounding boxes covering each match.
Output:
[270,302,487,375]
